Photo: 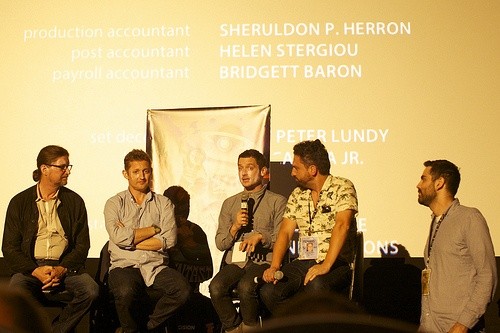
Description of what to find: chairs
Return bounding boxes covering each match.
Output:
[220,247,264,333]
[98,241,168,333]
[288,229,363,303]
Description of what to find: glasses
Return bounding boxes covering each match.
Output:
[45,163,73,172]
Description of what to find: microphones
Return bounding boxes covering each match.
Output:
[254,271,284,283]
[241,191,249,232]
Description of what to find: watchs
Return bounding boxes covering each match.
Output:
[152,223,160,234]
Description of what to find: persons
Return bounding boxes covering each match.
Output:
[209,149,289,332]
[105,149,189,333]
[260,137,358,316]
[1,144,100,333]
[417,159,498,333]
